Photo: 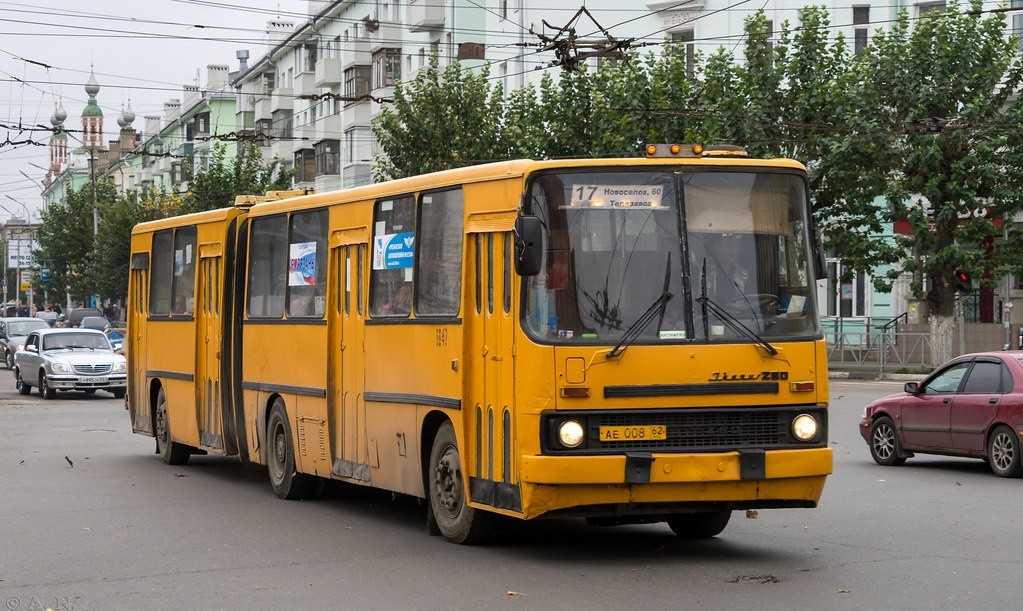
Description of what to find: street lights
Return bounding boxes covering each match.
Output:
[0,120,117,319]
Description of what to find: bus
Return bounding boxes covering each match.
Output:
[122,142,832,551]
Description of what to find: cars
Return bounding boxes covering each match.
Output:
[857,350,1022,477]
[12,328,127,400]
[0,299,126,371]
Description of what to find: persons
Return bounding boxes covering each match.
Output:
[289,286,322,320]
[174,295,189,315]
[1,300,122,325]
[52,313,71,329]
[698,236,782,319]
[381,279,434,319]
